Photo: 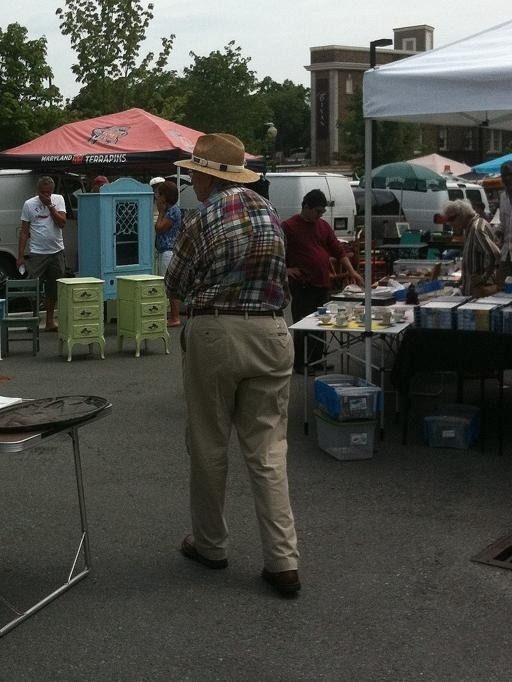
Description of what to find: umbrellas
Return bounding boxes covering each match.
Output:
[359,160,446,222]
[471,154,512,174]
[407,153,471,177]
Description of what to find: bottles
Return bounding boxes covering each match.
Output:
[19,264,25,275]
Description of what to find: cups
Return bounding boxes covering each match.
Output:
[317,304,407,327]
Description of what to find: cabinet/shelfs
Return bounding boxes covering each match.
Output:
[55,277,109,362]
[117,275,172,357]
[76,177,154,320]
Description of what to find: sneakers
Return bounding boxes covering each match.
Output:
[294,365,315,375]
[314,360,335,372]
[45,320,58,330]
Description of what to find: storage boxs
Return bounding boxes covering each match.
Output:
[424,403,482,450]
[311,407,380,460]
[314,373,383,421]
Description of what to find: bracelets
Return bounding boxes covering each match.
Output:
[48,204,56,210]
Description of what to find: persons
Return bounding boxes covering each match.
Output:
[433,159,512,297]
[155,181,182,328]
[16,176,66,332]
[165,133,301,594]
[281,189,365,376]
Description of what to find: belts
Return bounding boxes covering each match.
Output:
[187,308,283,318]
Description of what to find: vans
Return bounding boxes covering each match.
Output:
[349,176,504,254]
[0,169,86,297]
[165,171,357,245]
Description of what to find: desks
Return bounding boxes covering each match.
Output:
[427,236,465,257]
[1,395,114,634]
[378,242,428,272]
[287,269,462,448]
[389,327,510,456]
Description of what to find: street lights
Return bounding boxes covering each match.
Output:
[369,37,394,166]
[261,122,277,173]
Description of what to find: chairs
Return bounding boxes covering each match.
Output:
[1,278,42,356]
[352,240,387,283]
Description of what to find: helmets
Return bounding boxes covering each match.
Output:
[149,177,166,186]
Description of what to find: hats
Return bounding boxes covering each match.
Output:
[173,132,261,183]
[94,175,108,186]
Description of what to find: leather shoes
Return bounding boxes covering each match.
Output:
[182,533,228,568]
[263,565,302,592]
